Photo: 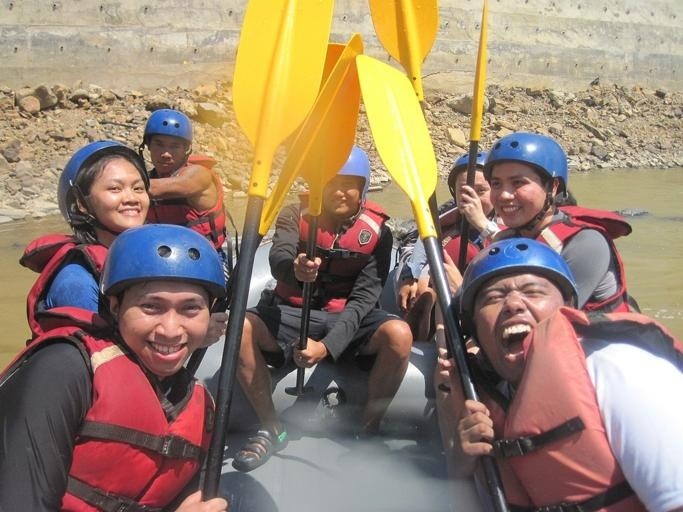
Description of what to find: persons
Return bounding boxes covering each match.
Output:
[428,132,642,477]
[0,224,228,512]
[139,108,238,287]
[447,236,683,512]
[396,151,509,342]
[232,147,412,474]
[18,140,228,349]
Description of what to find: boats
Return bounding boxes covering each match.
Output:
[180,225,546,512]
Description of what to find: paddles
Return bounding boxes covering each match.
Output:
[284,43,360,396]
[366,0,448,267]
[184,36,363,376]
[356,55,510,512]
[458,1,490,347]
[200,1,338,502]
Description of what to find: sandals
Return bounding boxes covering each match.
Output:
[230,417,292,476]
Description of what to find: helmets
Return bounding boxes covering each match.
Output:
[54,138,151,231]
[484,132,569,197]
[141,107,194,144]
[97,221,227,301]
[447,151,492,192]
[456,235,580,336]
[336,145,371,205]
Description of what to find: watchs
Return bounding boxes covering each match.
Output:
[480,220,497,238]
